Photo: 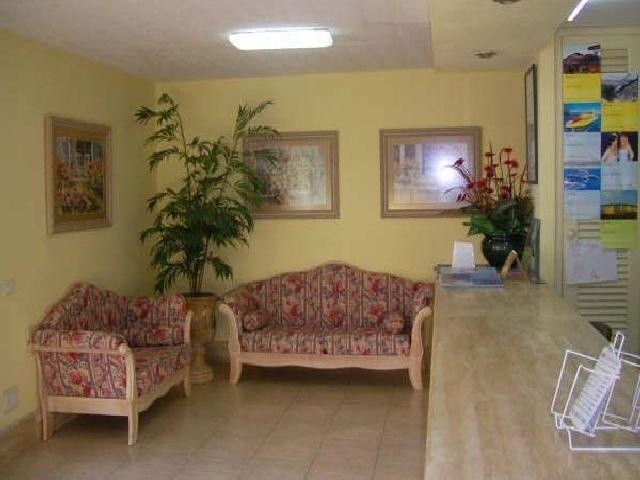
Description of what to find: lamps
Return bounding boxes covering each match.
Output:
[228,29,333,50]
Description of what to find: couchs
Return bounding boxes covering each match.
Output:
[30,283,194,449]
[218,262,434,390]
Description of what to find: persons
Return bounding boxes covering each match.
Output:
[601,136,618,162]
[613,131,635,162]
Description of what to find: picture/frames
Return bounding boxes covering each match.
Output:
[524,64,537,183]
[241,124,484,221]
[43,113,115,238]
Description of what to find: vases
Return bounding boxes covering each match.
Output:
[482,234,532,270]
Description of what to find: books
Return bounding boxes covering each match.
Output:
[439,263,505,288]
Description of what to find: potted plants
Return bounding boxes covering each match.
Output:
[137,92,280,383]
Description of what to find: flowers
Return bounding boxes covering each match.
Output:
[440,144,535,239]
